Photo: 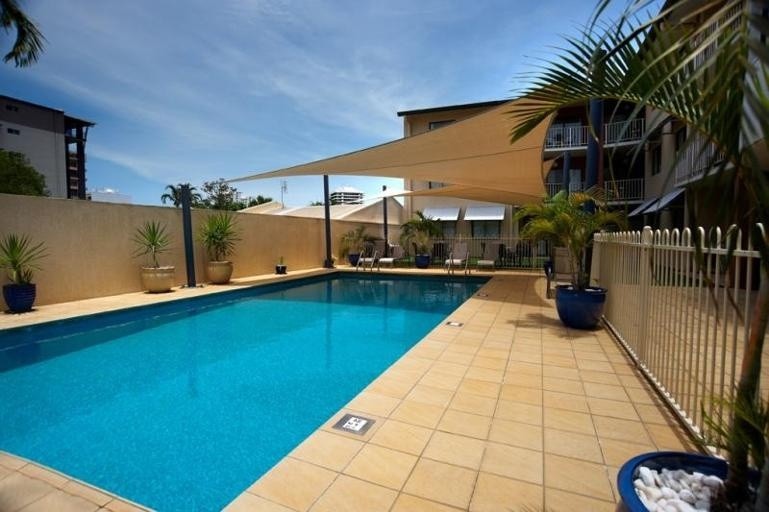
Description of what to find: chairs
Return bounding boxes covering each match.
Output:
[378,245,398,267]
[446,241,498,270]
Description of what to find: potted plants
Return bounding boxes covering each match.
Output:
[335,224,377,266]
[503,1,768,512]
[129,210,244,291]
[1,209,51,313]
[275,257,286,275]
[398,209,445,268]
